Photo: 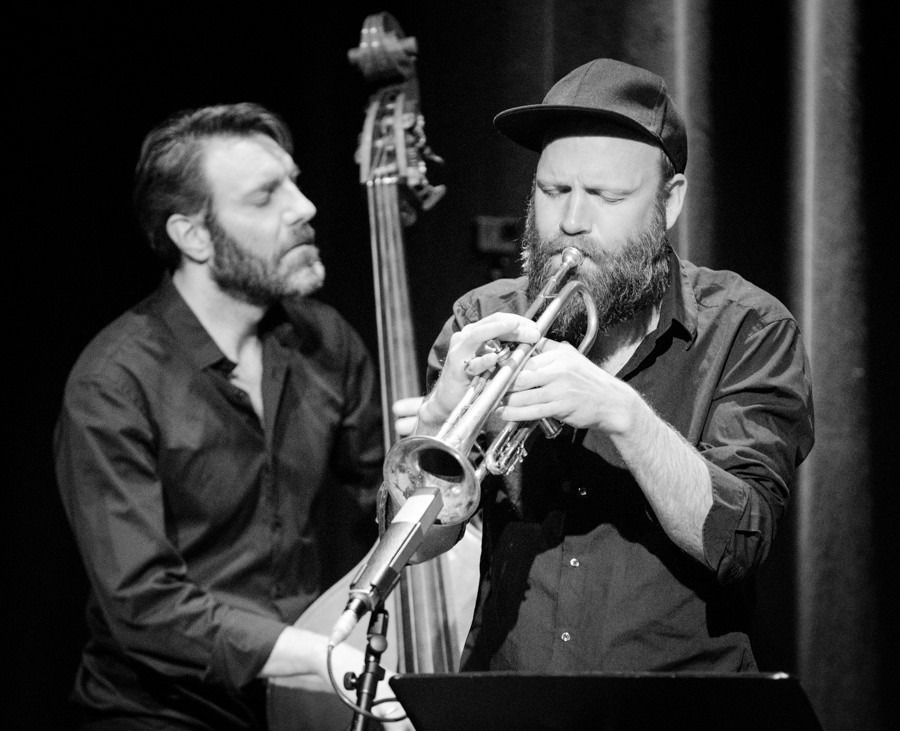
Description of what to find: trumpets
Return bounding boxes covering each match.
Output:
[382,244,599,528]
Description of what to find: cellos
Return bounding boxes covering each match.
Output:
[263,10,483,731]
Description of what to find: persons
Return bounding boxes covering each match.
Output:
[54,102,424,731]
[408,57,815,674]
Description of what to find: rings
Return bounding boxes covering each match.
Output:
[464,360,469,371]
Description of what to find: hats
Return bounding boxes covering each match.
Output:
[493,58,688,173]
